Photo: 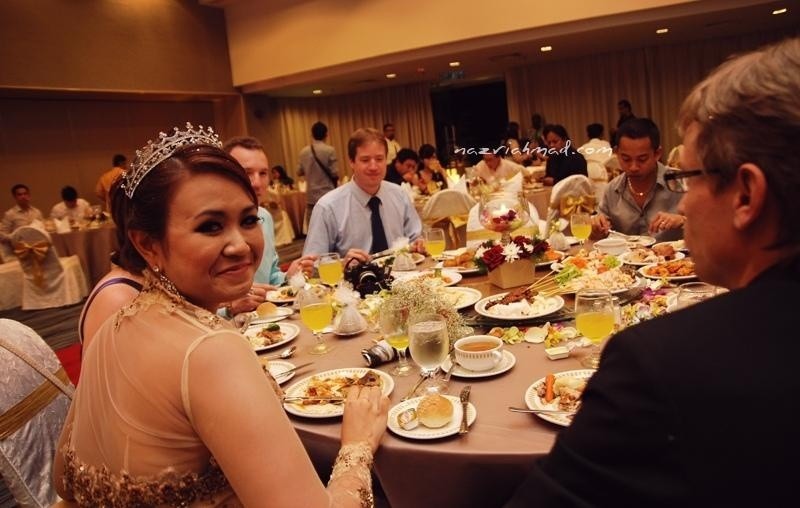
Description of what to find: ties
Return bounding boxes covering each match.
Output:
[367,196,389,255]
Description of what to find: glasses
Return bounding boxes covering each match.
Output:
[664,167,704,194]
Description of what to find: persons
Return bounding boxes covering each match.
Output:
[456,163,466,177]
[527,138,548,167]
[576,123,613,161]
[1,185,43,242]
[588,118,684,242]
[96,154,129,213]
[507,39,800,508]
[418,143,448,196]
[384,147,418,185]
[301,128,429,278]
[296,121,340,232]
[528,113,543,138]
[500,121,530,163]
[535,125,588,187]
[466,150,530,190]
[269,165,294,187]
[50,186,90,220]
[216,136,317,318]
[78,169,147,365]
[382,124,400,165]
[52,122,390,508]
[446,161,457,177]
[609,99,636,137]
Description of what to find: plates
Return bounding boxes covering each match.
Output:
[387,396,477,440]
[618,250,684,265]
[392,270,462,292]
[241,322,301,351]
[525,367,601,429]
[369,251,423,269]
[447,286,482,312]
[246,306,293,325]
[279,366,395,419]
[267,361,295,384]
[265,283,327,304]
[474,289,565,321]
[536,249,566,268]
[624,234,657,248]
[435,255,487,273]
[544,234,580,247]
[551,256,625,275]
[652,240,698,253]
[442,349,516,378]
[639,261,707,281]
[444,245,471,257]
[574,275,641,297]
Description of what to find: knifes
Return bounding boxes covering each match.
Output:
[272,361,315,380]
[369,248,395,257]
[283,395,346,403]
[252,312,294,321]
[460,384,472,436]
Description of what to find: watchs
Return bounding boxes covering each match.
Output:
[225,301,232,316]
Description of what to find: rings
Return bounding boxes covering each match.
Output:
[662,222,665,225]
[250,286,255,294]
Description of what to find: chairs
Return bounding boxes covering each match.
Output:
[1,318,86,508]
[425,189,476,250]
[11,225,82,308]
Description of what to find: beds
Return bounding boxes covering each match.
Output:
[217,231,731,508]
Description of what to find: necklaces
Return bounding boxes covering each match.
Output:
[627,176,657,197]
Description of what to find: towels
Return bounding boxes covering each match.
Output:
[445,176,469,192]
[297,181,306,193]
[402,181,414,201]
[53,217,72,233]
[31,219,44,229]
[506,171,523,193]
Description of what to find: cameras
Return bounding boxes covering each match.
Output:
[341,256,393,299]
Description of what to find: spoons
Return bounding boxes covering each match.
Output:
[443,352,463,381]
[261,345,298,360]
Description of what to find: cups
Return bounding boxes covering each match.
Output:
[571,212,592,258]
[318,251,344,307]
[574,288,615,370]
[453,334,504,373]
[409,313,450,398]
[676,281,716,309]
[379,299,420,376]
[297,288,336,356]
[594,237,625,255]
[479,189,531,247]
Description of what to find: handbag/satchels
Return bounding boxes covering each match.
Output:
[309,143,340,187]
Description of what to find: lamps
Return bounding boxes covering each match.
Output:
[313,89,322,95]
[540,46,553,52]
[449,62,460,67]
[655,29,668,34]
[772,8,787,15]
[385,73,396,79]
